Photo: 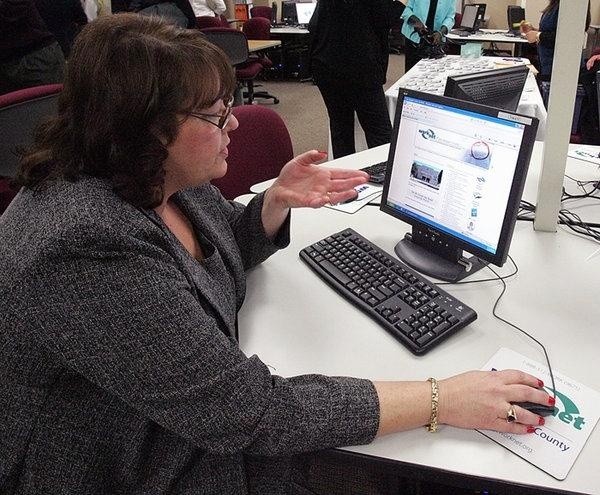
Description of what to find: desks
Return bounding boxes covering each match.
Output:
[270,24,309,80]
[443,29,529,57]
[588,25,600,54]
[383,54,547,142]
[229,141,600,495]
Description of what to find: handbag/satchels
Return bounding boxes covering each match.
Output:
[410,28,446,60]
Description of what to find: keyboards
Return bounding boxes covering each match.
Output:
[359,159,388,183]
[299,228,477,355]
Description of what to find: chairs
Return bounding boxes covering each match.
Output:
[1,6,284,110]
[210,103,293,198]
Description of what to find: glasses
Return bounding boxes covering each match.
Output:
[176,93,235,130]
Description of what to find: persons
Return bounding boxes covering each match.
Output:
[0,0,229,95]
[586,53,600,71]
[518,0,592,142]
[399,0,457,75]
[1,11,556,494]
[306,0,411,161]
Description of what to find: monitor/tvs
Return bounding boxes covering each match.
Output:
[379,88,540,280]
[507,5,525,35]
[295,2,317,29]
[444,66,529,112]
[281,0,301,22]
[460,5,481,31]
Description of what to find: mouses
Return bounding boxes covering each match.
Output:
[511,386,554,415]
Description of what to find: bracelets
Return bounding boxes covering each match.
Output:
[425,377,440,434]
[536,31,541,42]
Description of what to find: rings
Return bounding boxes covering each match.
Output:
[506,404,518,426]
